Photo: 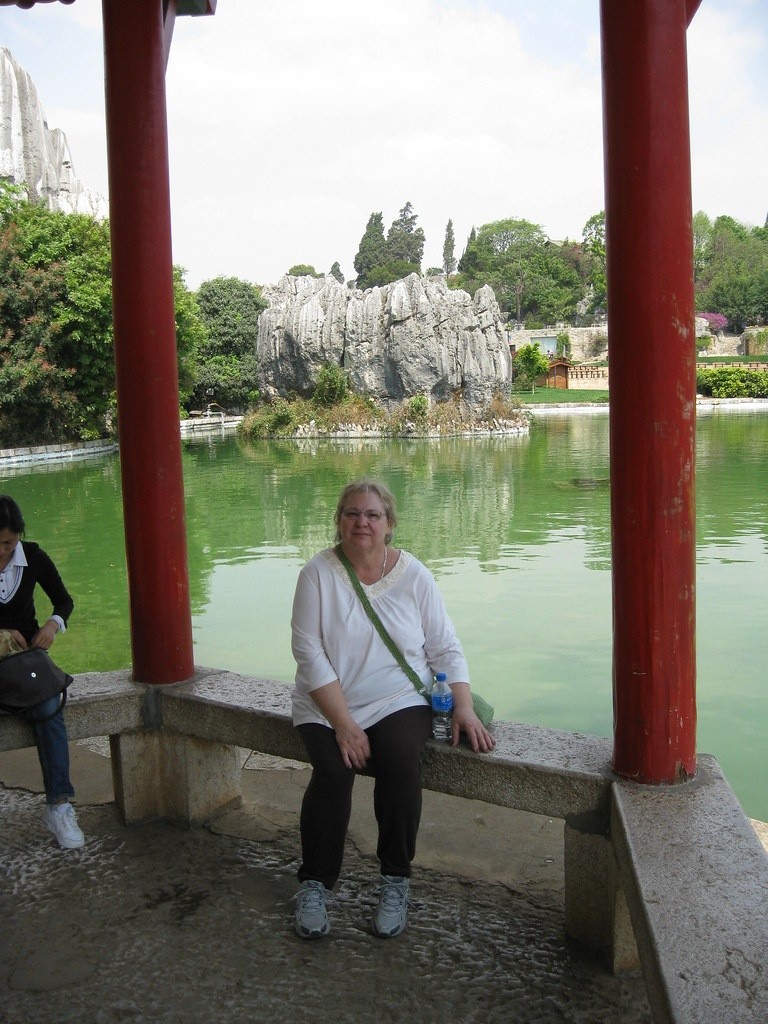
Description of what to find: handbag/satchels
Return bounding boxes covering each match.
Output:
[0,648,73,722]
[426,690,494,728]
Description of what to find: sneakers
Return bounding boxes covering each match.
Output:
[286,880,334,939]
[369,874,418,938]
[42,804,85,848]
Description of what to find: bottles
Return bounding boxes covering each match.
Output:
[431,674,454,741]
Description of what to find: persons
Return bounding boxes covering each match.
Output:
[287,480,497,942]
[0,493,86,850]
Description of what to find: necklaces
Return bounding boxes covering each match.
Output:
[380,544,387,581]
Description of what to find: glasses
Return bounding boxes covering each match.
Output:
[341,507,388,522]
